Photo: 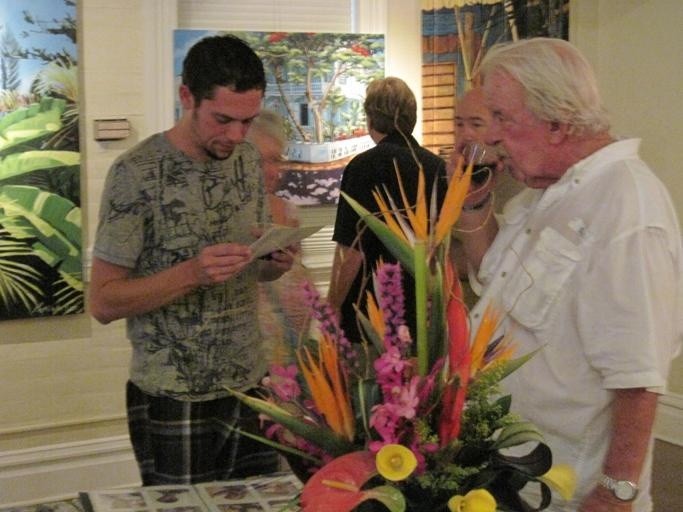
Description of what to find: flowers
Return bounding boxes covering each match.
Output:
[216,152,575,512]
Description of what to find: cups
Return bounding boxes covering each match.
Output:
[458,141,501,181]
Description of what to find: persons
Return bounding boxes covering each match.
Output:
[325,76,447,355]
[448,36,682,512]
[451,87,526,312]
[252,109,305,370]
[85,35,299,489]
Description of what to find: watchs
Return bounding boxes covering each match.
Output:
[597,473,641,503]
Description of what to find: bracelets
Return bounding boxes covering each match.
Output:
[461,191,491,210]
